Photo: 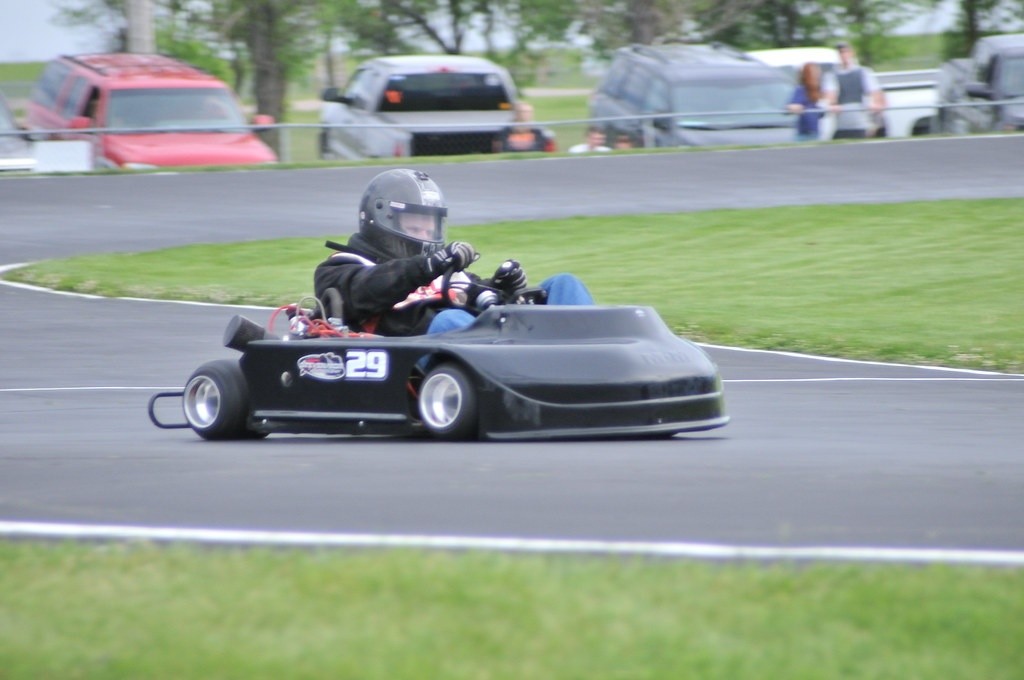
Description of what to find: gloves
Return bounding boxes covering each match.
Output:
[492,259,529,295]
[429,240,475,274]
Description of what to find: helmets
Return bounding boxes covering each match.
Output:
[348,167,449,263]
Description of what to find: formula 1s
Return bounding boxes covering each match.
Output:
[146,251,738,440]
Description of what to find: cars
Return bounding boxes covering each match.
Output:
[1,98,34,169]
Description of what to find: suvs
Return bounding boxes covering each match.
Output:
[315,54,556,162]
[932,31,1024,135]
[24,52,274,167]
[862,64,950,138]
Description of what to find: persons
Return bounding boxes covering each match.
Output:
[312,168,595,382]
[784,60,833,142]
[566,126,614,155]
[492,102,558,154]
[823,41,884,141]
[612,130,645,151]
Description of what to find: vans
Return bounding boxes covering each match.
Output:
[589,42,826,148]
[741,46,886,138]
